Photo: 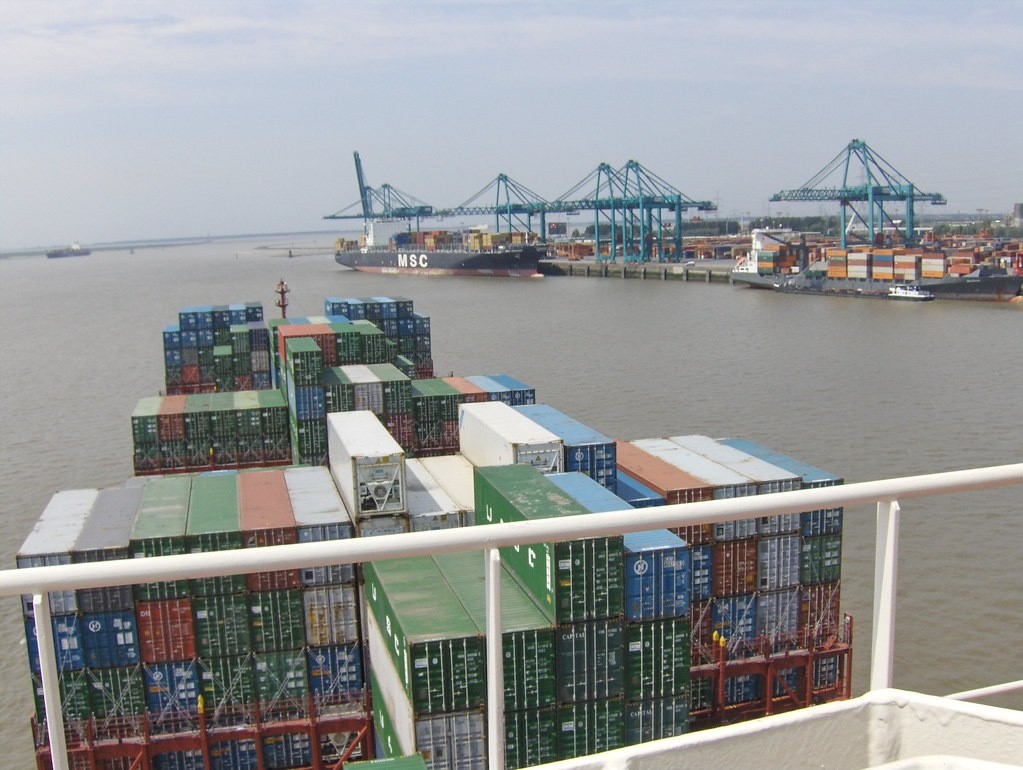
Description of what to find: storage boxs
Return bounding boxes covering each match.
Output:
[387,229,733,263]
[16,295,844,770]
[759,235,1023,283]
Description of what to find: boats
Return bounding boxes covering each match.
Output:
[727,267,1023,304]
[886,283,936,302]
[334,237,550,278]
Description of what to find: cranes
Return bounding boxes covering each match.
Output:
[767,137,948,250]
[321,150,718,266]
